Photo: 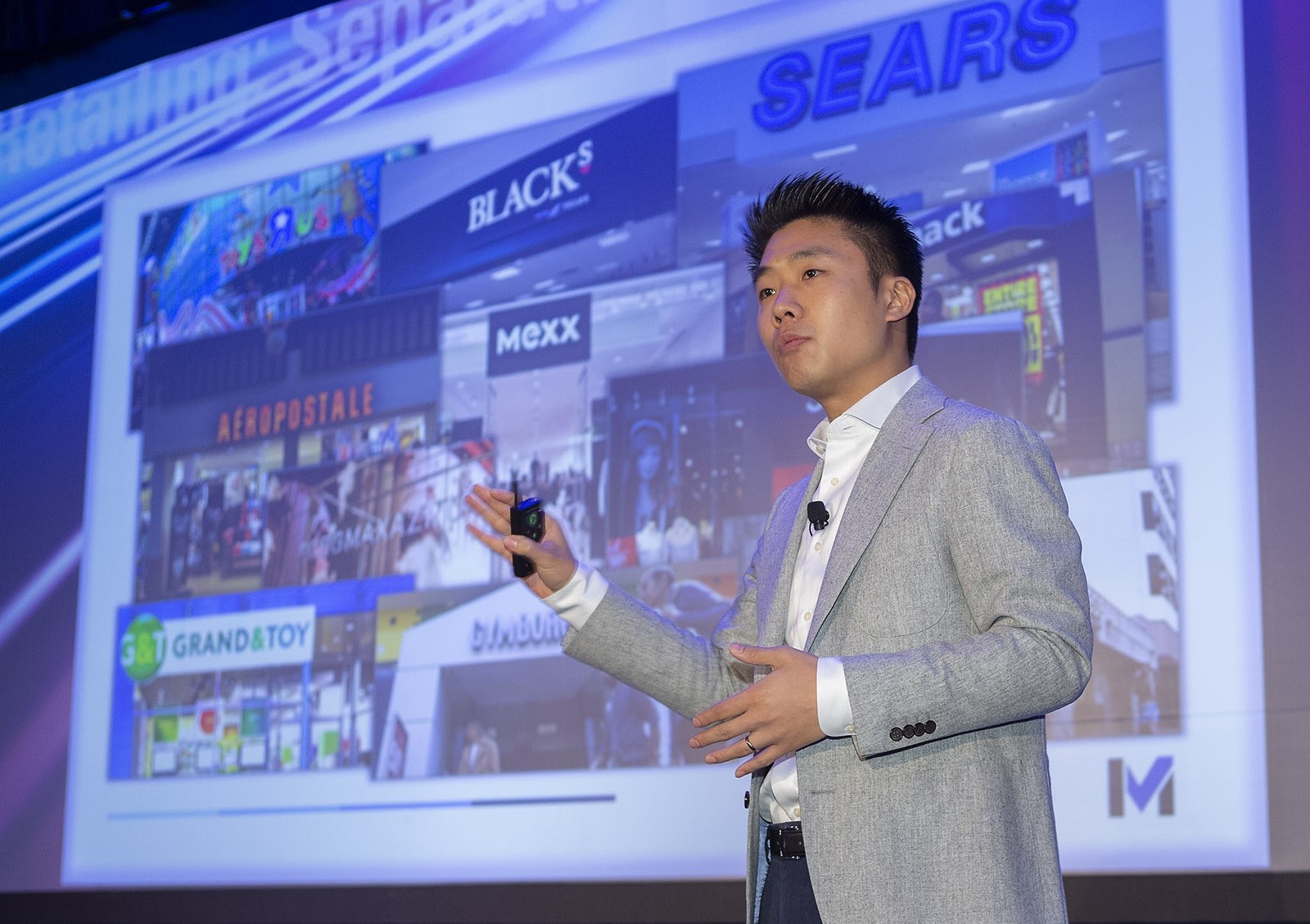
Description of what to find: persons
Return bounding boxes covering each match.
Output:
[620,419,672,533]
[640,569,732,639]
[458,714,500,776]
[464,166,1094,924]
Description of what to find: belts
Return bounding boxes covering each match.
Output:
[767,825,806,860]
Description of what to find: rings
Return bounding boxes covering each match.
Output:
[745,736,759,752]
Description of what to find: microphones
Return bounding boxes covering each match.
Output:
[807,501,829,530]
